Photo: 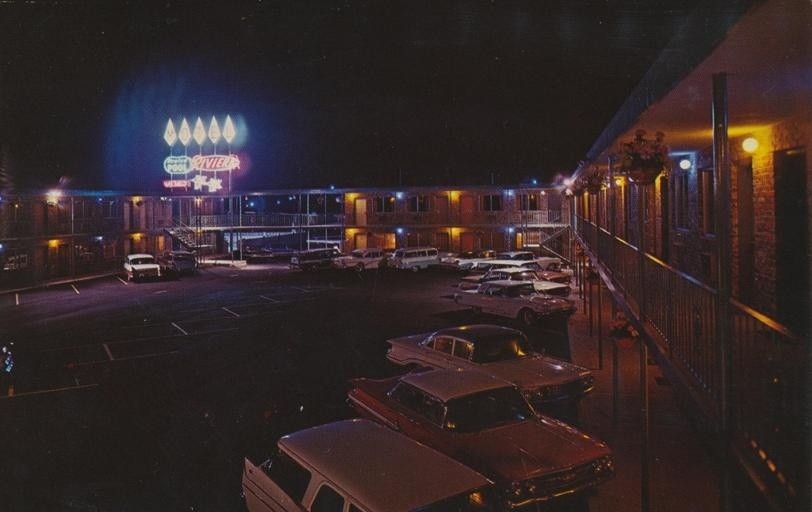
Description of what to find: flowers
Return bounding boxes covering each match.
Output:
[620,129,677,180]
[578,165,612,191]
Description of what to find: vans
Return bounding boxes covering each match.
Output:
[3,253,27,271]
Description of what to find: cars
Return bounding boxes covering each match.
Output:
[121,254,161,280]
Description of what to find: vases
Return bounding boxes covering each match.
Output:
[588,186,599,195]
[630,168,661,186]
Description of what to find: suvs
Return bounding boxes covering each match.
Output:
[158,249,196,279]
